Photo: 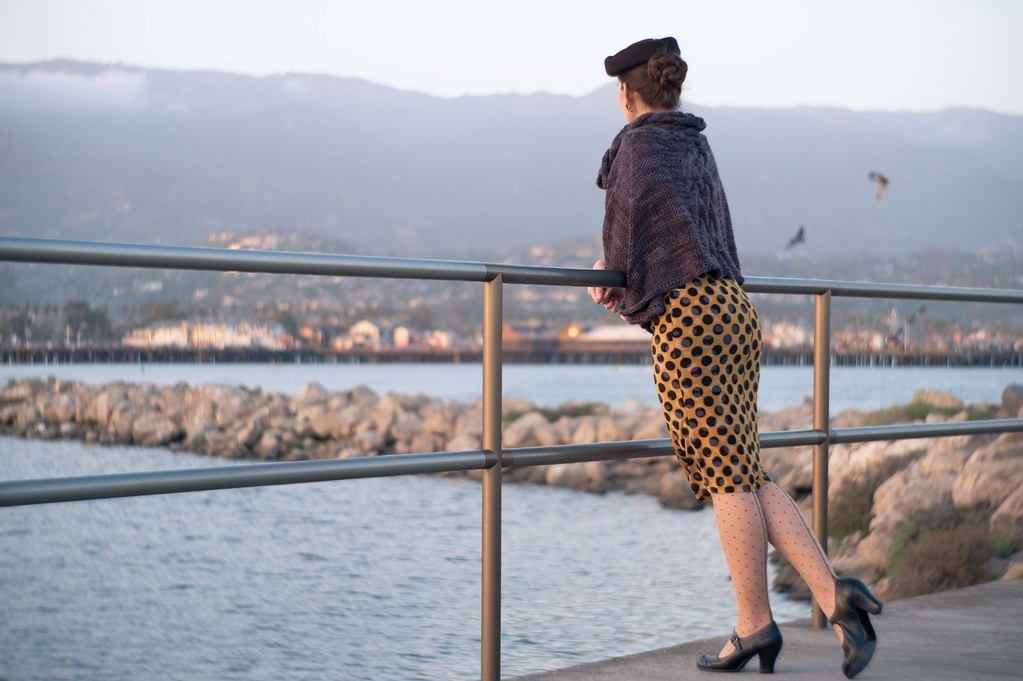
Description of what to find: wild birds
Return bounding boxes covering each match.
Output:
[782,225,809,249]
[882,304,926,353]
[867,171,889,199]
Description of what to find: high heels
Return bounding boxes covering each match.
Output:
[828,577,884,678]
[696,621,783,676]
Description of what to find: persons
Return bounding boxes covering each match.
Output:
[588,37,883,678]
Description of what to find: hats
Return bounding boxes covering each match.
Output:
[604,34,681,76]
